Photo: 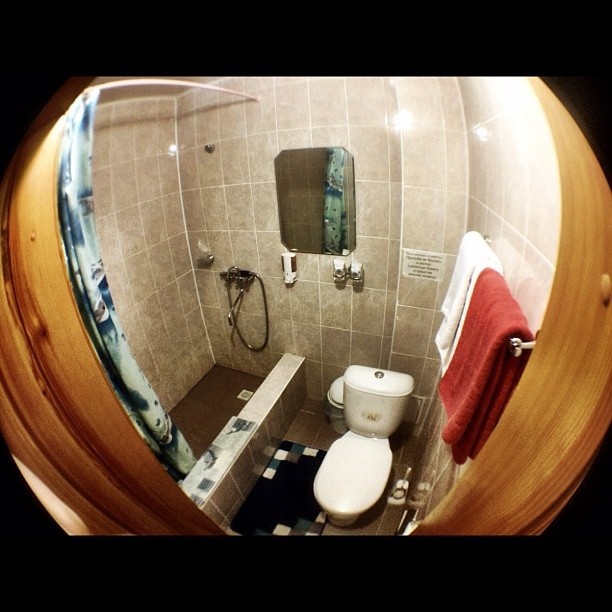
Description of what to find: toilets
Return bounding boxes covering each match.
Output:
[313,365,415,526]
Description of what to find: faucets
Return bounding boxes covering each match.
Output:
[228,283,242,326]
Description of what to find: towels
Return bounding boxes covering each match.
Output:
[434,229,503,377]
[435,269,536,464]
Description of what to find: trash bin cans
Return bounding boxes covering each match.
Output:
[326,376,349,434]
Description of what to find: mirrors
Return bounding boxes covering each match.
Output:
[274,147,356,256]
